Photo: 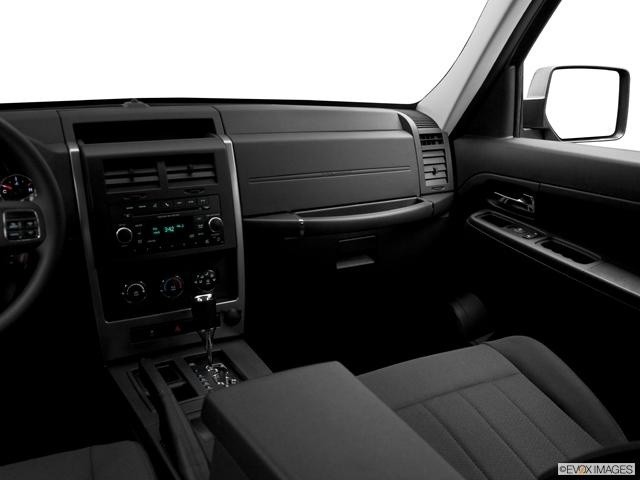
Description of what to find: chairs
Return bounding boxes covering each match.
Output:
[0,439,159,480]
[355,335,639,480]
